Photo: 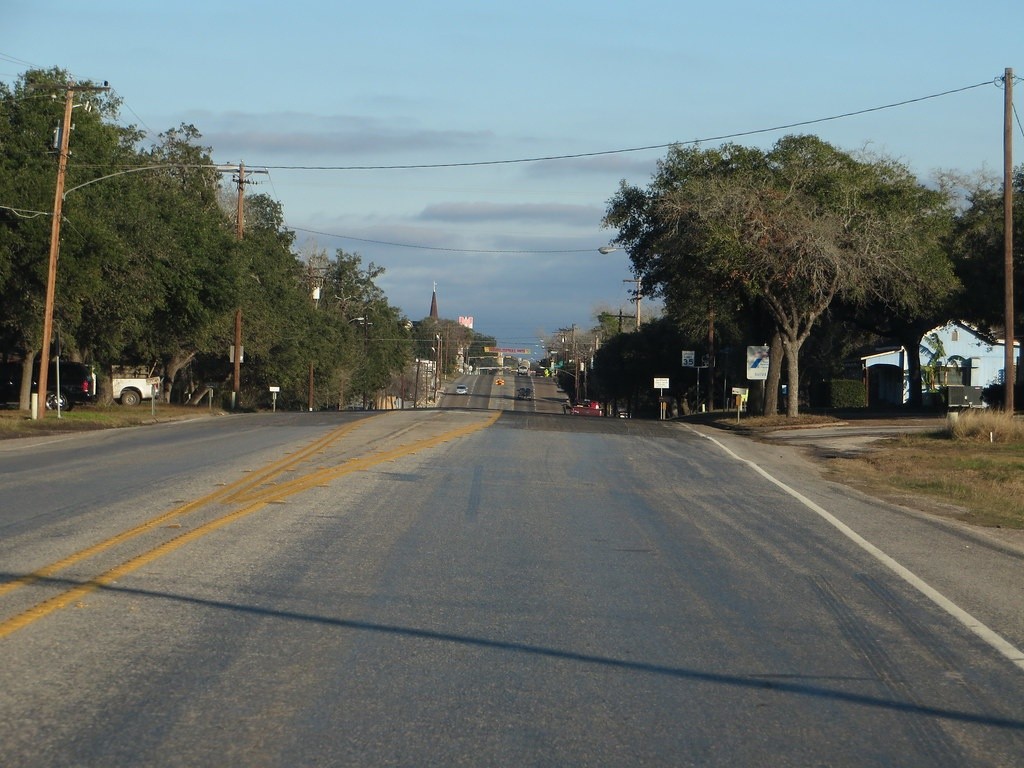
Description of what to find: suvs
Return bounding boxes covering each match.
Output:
[517,366,528,376]
[0,361,93,411]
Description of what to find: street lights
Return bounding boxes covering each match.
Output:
[598,246,640,332]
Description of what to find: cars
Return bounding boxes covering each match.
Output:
[517,387,533,401]
[456,384,468,395]
